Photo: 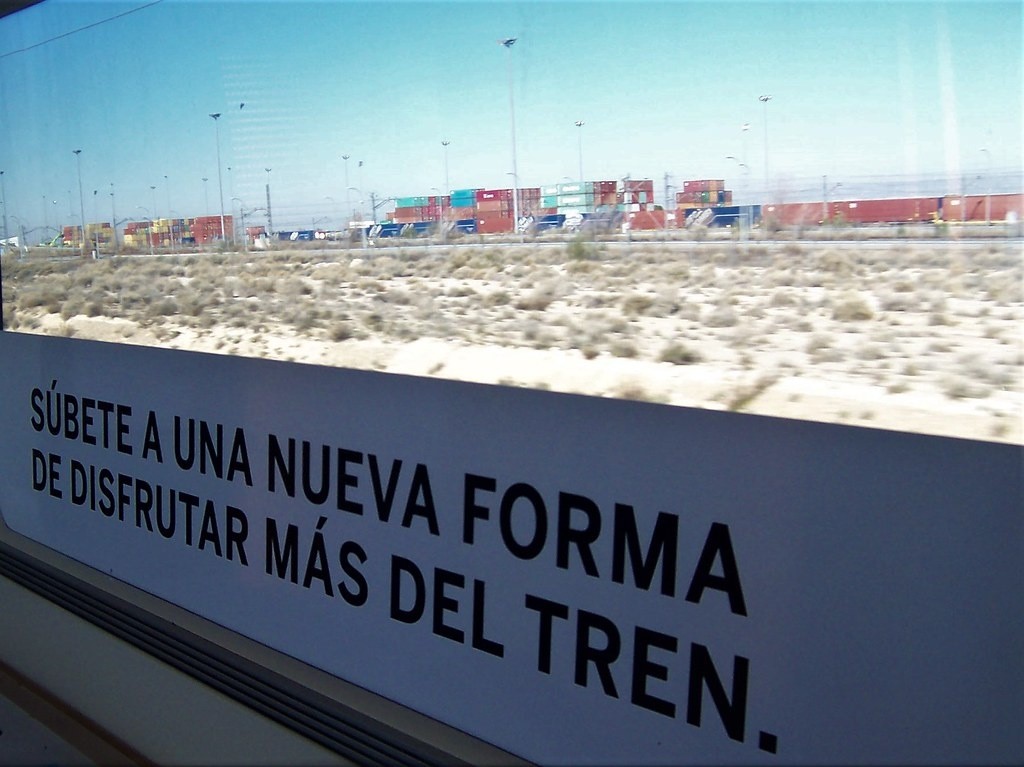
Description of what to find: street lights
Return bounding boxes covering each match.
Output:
[498,36,521,234]
[575,119,584,181]
[106,174,178,255]
[0,170,9,255]
[724,94,773,201]
[342,153,366,223]
[72,149,85,247]
[209,112,226,241]
[442,140,452,193]
[265,167,274,234]
